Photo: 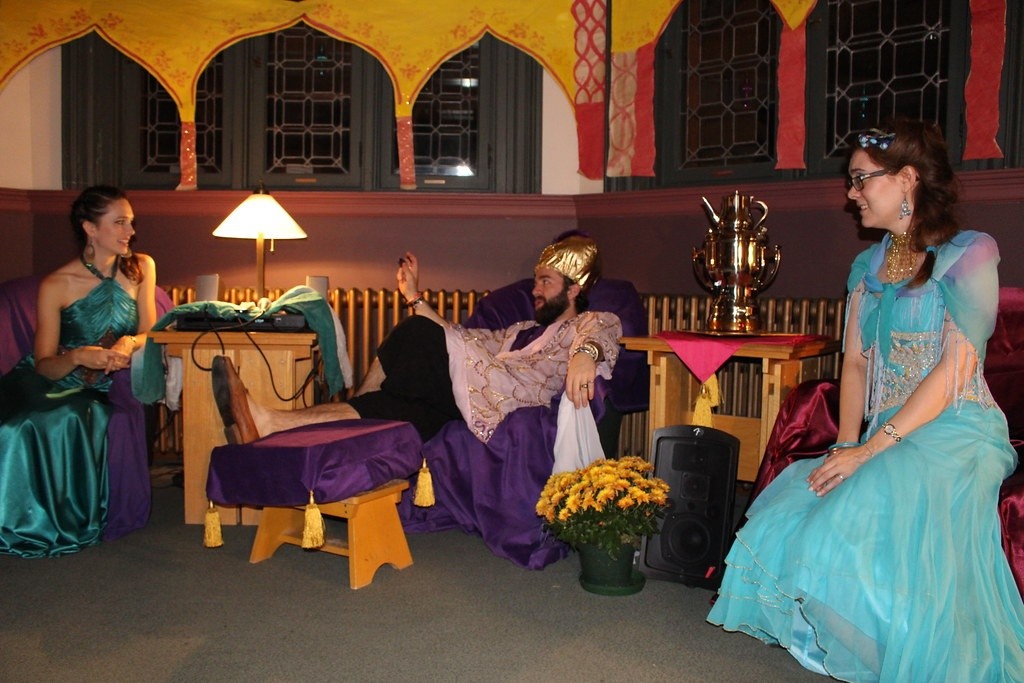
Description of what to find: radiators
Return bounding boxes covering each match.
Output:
[158,287,845,456]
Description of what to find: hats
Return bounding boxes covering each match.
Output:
[533,235,599,283]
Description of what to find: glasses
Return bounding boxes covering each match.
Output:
[847,169,899,191]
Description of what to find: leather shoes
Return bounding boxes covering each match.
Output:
[212,355,260,445]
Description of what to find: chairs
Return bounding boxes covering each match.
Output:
[396,276,650,571]
[747,284,1024,606]
[0,268,175,545]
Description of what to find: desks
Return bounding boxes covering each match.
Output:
[145,330,319,526]
[616,337,840,485]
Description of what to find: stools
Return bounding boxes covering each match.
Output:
[249,479,414,590]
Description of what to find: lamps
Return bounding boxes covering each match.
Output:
[212,177,307,300]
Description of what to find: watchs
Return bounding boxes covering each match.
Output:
[881,421,902,442]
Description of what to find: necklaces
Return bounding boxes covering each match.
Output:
[885,225,919,284]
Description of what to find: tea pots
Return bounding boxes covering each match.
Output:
[700,189,769,231]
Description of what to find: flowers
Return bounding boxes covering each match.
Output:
[535,456,671,561]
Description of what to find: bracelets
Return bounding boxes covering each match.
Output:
[573,342,598,360]
[863,443,874,457]
[131,336,136,343]
[405,291,425,307]
[829,441,861,451]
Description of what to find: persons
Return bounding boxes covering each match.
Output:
[706,119,1024,683]
[0,183,157,555]
[211,229,623,446]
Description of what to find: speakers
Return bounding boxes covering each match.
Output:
[637,424,741,590]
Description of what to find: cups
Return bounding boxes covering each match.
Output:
[306,276,328,301]
[195,273,219,301]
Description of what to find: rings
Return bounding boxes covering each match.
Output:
[588,380,594,383]
[832,448,839,455]
[580,384,588,388]
[838,474,845,480]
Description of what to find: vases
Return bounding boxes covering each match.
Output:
[574,542,646,595]
